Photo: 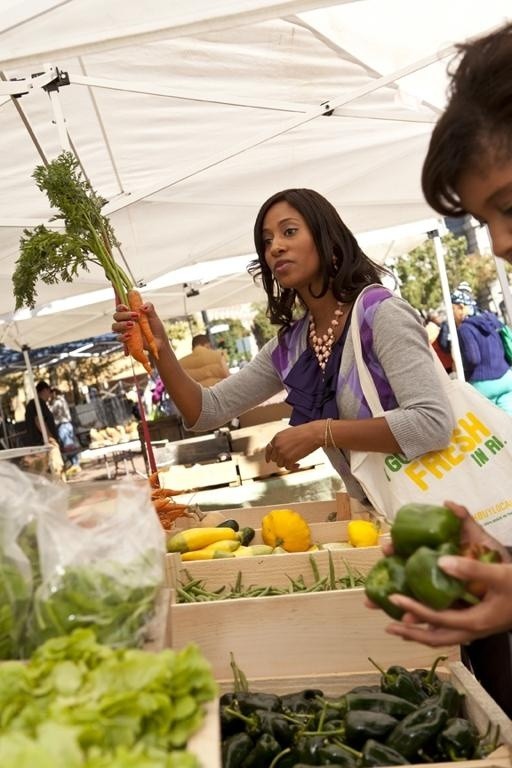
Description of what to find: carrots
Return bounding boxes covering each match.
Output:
[116,289,160,380]
[64,466,207,530]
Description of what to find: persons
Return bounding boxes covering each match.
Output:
[443,274,512,411]
[108,184,511,721]
[47,388,85,475]
[362,23,512,651]
[26,379,59,447]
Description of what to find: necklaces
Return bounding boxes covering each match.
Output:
[304,304,345,368]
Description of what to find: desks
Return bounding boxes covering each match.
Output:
[75,440,155,482]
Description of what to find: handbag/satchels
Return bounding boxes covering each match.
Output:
[351,378,512,546]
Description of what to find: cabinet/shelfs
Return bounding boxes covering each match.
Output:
[148,485,512,768]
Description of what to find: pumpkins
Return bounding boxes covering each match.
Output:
[167,507,383,562]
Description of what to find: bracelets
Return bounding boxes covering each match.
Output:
[328,417,338,450]
[324,416,329,451]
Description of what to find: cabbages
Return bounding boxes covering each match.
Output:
[1,625,219,767]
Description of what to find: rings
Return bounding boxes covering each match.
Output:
[268,442,275,450]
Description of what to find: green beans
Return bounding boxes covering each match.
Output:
[172,544,367,604]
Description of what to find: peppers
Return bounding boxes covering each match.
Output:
[362,502,504,619]
[216,643,504,768]
